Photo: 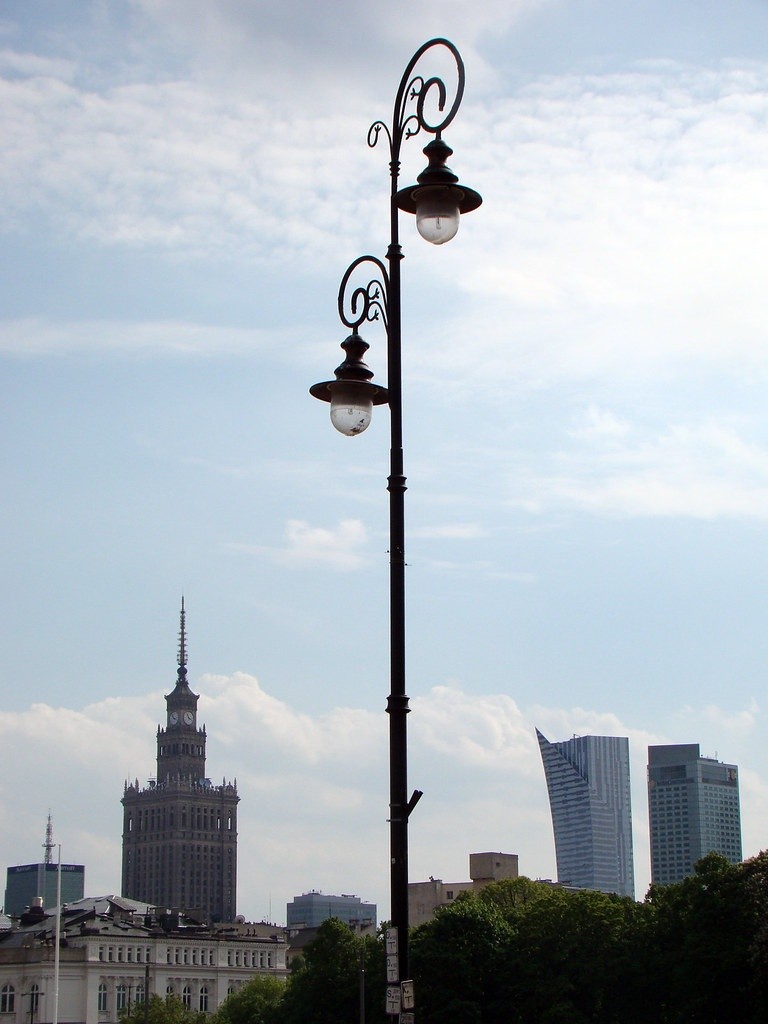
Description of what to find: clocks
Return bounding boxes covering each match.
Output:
[168,711,179,725]
[183,710,194,725]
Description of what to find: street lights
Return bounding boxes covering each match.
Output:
[307,35,484,1023]
